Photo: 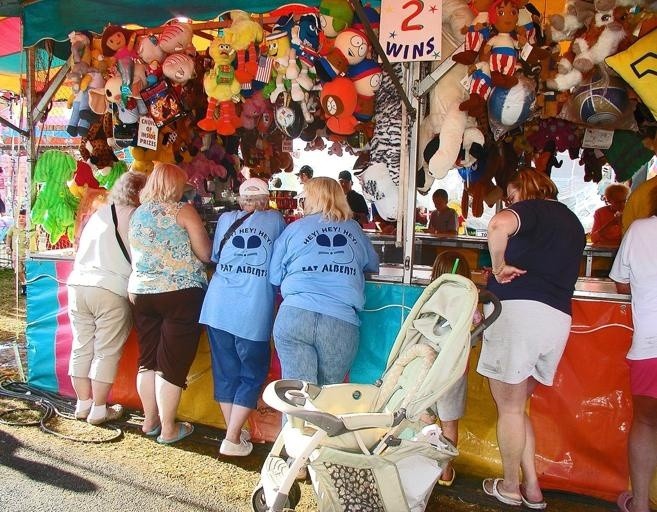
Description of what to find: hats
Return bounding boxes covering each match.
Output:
[238,178,270,196]
[338,169,352,180]
[295,165,314,177]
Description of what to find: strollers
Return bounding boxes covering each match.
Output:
[246,276,503,512]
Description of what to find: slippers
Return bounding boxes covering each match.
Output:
[145,412,162,436]
[437,464,456,488]
[482,476,524,508]
[75,405,91,420]
[86,403,124,425]
[617,491,634,512]
[519,481,549,511]
[156,422,195,444]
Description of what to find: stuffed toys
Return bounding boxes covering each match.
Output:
[27,0,656,249]
[28,1,657,245]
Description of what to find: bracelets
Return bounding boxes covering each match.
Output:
[491,261,505,275]
[6,246,10,249]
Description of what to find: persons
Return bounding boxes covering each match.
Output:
[4,208,28,295]
[416,249,483,486]
[197,178,287,457]
[66,169,148,426]
[266,176,380,481]
[589,183,631,278]
[124,162,215,444]
[605,173,656,510]
[473,165,585,510]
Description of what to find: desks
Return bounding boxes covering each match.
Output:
[26,230,656,511]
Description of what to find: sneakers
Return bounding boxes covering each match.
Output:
[20,282,27,296]
[219,438,255,457]
[240,429,251,442]
